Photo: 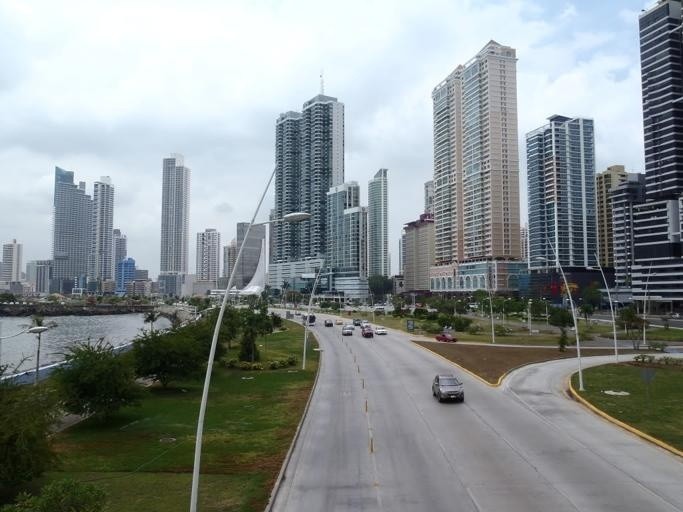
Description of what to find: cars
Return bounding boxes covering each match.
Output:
[325,319,333,326]
[435,333,457,342]
[354,319,386,337]
[336,319,355,335]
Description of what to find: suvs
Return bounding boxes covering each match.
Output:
[432,375,464,402]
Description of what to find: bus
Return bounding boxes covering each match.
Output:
[302,314,315,326]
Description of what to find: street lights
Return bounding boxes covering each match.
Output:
[188,212,310,510]
[0,326,48,341]
[536,256,618,391]
[642,273,657,344]
